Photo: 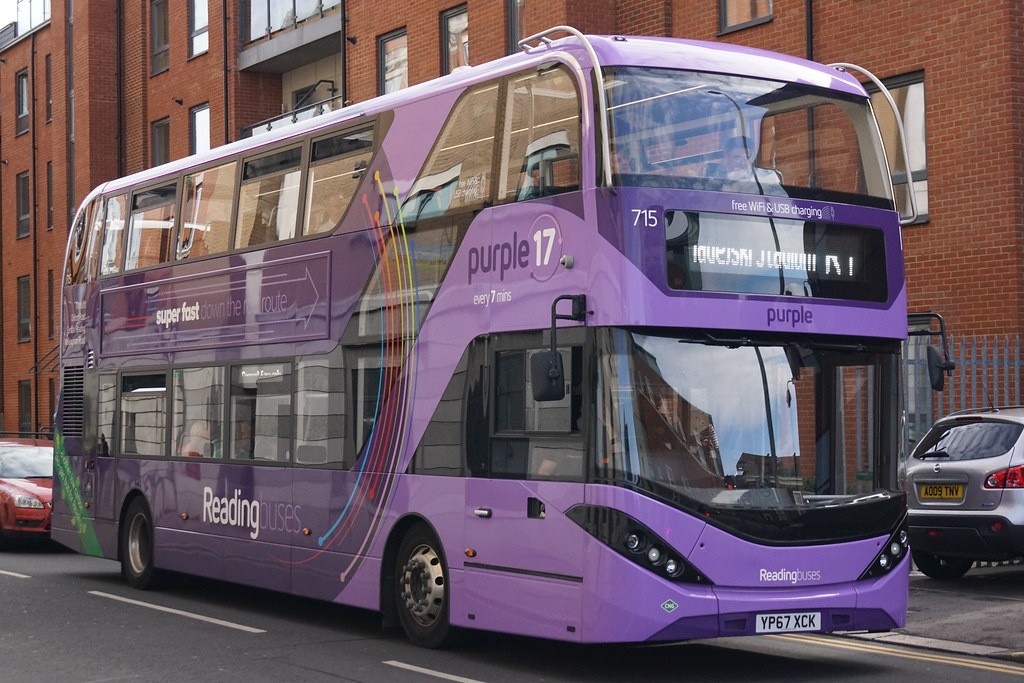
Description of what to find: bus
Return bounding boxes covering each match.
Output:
[48,24,956,651]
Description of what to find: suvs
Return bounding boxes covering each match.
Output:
[898,404,1024,580]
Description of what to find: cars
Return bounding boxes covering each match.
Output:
[0,436,54,552]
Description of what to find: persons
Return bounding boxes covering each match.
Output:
[317,191,347,233]
[182,422,214,457]
[710,136,788,197]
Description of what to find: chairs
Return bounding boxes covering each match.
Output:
[142,206,285,266]
